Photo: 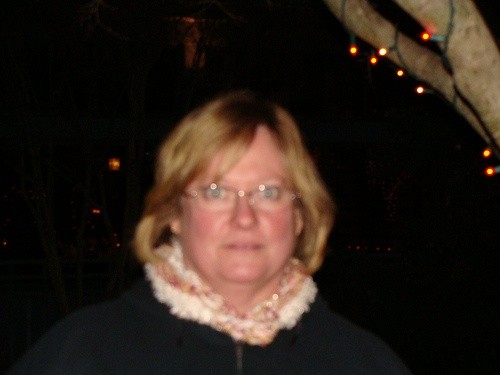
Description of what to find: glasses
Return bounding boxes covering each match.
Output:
[182,183,306,212]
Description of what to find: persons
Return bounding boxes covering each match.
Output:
[35,94,404,374]
